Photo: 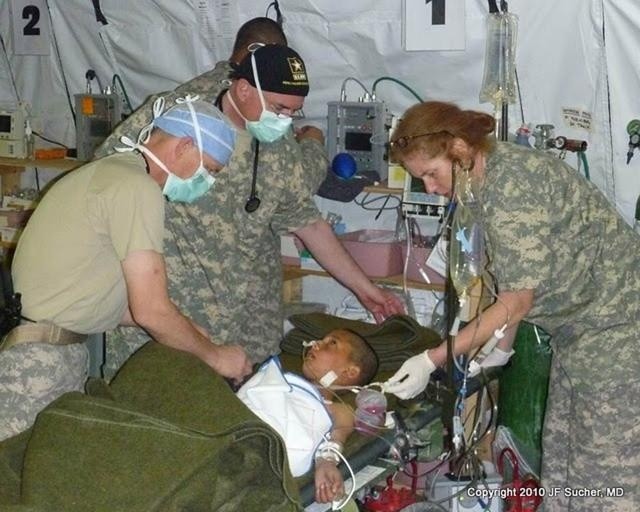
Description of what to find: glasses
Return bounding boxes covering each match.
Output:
[389,129,454,146]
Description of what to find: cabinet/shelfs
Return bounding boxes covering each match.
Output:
[277,182,446,302]
[1,157,87,272]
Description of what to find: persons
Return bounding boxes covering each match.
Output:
[231,326,379,504]
[2,96,260,451]
[97,44,387,396]
[167,15,289,105]
[373,101,640,512]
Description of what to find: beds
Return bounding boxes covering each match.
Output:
[0,358,505,511]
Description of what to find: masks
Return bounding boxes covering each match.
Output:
[162,170,215,203]
[243,109,292,144]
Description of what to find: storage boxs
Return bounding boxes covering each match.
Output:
[399,235,448,287]
[340,228,405,277]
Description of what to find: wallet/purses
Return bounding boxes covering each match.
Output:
[497,446,544,512]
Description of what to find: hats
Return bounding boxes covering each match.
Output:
[232,43,310,100]
